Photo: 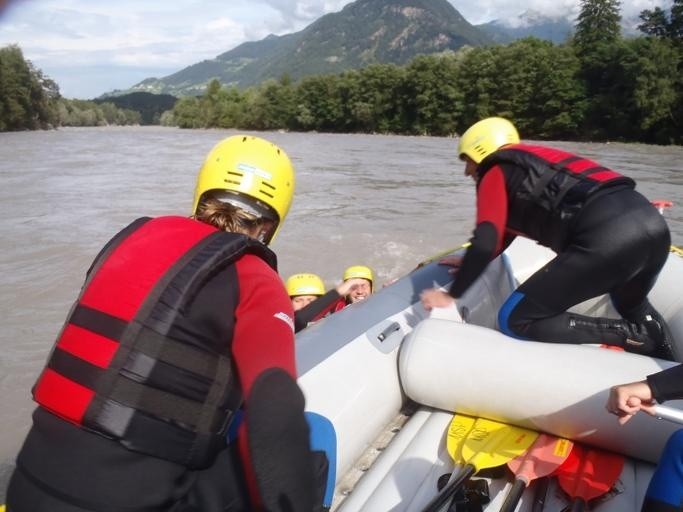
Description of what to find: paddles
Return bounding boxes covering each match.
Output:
[419,413,626,512]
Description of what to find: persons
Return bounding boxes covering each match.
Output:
[331,265,398,313]
[608,365,683,512]
[285,273,366,334]
[418,116,676,361]
[6,135,337,512]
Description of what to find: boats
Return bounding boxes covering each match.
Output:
[283,231,682,512]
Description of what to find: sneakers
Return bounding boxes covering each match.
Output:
[616,312,679,363]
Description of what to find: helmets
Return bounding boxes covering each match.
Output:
[458,116,521,164]
[343,265,375,295]
[285,273,326,297]
[190,134,296,248]
